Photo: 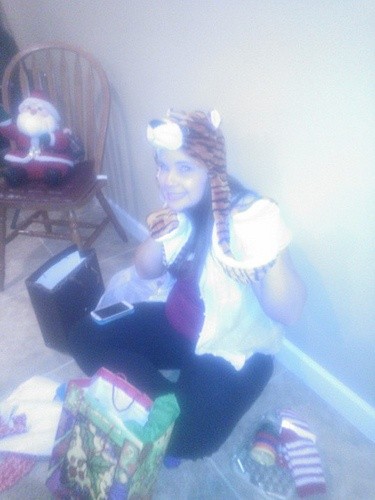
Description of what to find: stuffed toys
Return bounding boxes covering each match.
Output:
[0,90,87,185]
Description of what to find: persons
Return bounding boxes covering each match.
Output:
[65,103,308,468]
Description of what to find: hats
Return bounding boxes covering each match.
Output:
[145,106,226,168]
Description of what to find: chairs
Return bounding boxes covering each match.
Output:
[0,42,128,292]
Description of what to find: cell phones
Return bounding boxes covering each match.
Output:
[89,300,135,326]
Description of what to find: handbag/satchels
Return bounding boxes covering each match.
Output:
[46,367,177,500]
[25,242,105,356]
